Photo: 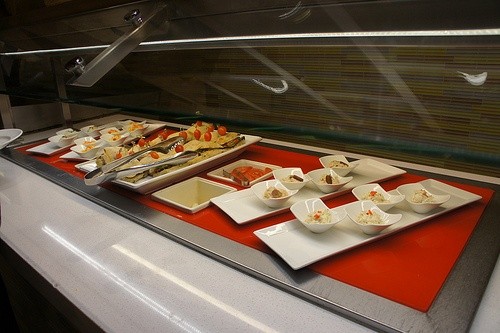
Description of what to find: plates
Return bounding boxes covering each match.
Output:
[76,123,262,195]
[210,157,407,226]
[25,121,130,156]
[205,158,283,187]
[252,178,482,271]
[0,128,23,150]
[59,125,167,162]
[150,176,236,214]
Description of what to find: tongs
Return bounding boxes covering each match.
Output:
[222,169,250,187]
[83,136,200,187]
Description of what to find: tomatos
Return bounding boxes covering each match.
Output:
[231,166,272,182]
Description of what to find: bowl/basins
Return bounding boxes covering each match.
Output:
[252,178,298,207]
[397,183,452,213]
[48,119,150,158]
[352,183,406,211]
[291,198,345,233]
[272,168,312,191]
[307,167,354,194]
[345,200,402,235]
[319,154,359,176]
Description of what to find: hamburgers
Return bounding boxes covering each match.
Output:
[97,121,247,181]
